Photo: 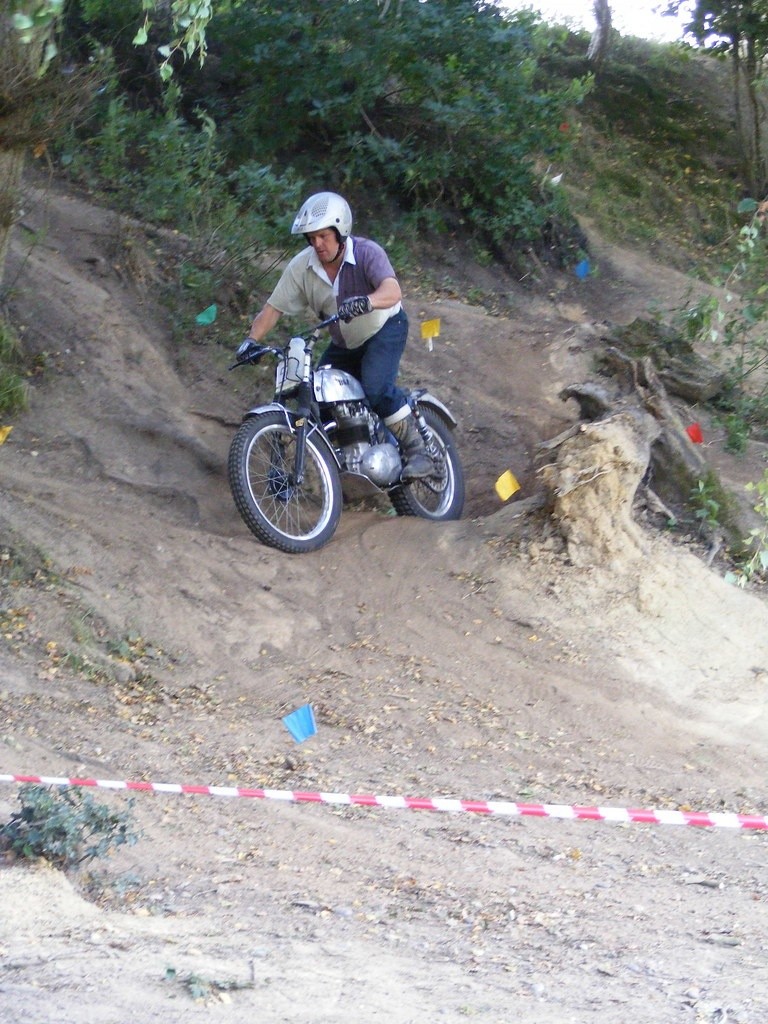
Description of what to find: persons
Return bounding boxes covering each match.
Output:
[236,192,436,484]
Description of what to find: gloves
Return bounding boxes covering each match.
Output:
[235,338,261,367]
[337,296,374,325]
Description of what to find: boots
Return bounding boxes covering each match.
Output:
[384,404,434,481]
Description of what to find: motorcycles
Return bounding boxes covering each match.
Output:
[225,301,465,554]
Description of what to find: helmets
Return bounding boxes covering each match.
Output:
[291,192,352,244]
